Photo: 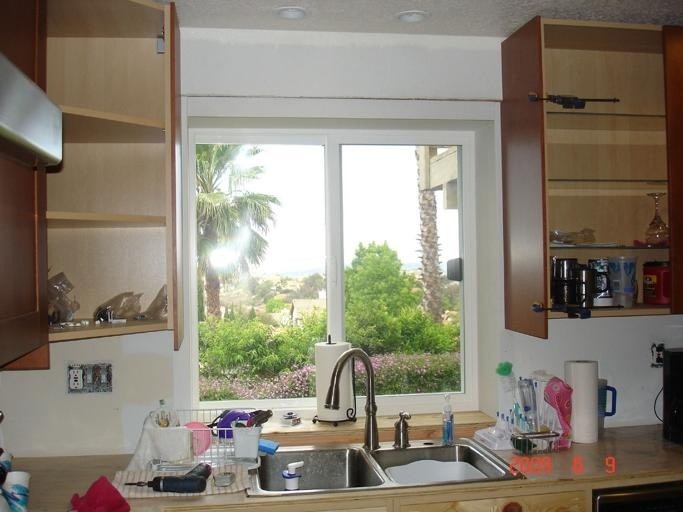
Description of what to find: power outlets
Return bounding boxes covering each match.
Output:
[65,361,112,395]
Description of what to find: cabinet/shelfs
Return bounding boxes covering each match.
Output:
[501,15,679,341]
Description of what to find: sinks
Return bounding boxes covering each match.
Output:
[243,443,384,496]
[370,435,525,488]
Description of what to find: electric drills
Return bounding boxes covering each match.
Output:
[125,462,211,493]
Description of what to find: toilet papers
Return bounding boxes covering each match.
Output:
[562,359,601,447]
[312,340,355,422]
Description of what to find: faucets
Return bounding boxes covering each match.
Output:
[323,346,381,451]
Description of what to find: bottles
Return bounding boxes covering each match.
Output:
[91,364,101,386]
[492,370,559,447]
[68,366,86,391]
[80,365,87,387]
[105,364,112,386]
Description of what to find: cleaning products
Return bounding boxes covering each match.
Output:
[156,399,179,428]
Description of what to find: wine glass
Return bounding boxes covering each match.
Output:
[644,191,670,244]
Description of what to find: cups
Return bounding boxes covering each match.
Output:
[0,470,32,512]
[0,451,14,472]
[553,254,668,309]
[598,378,617,436]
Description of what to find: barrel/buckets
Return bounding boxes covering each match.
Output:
[232,421,263,460]
